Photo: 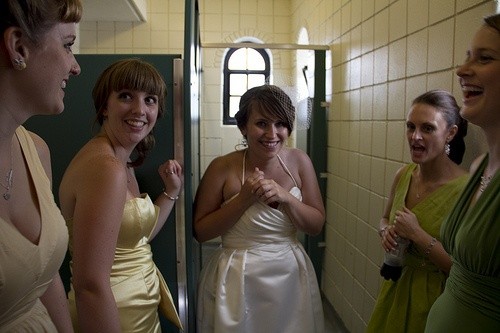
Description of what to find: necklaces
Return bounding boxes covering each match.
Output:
[0,136,15,200]
[380,228,384,236]
[480,175,493,192]
[413,179,426,199]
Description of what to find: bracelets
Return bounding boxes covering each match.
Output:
[164,191,179,201]
[423,238,436,257]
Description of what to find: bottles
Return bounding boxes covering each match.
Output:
[380,234,410,281]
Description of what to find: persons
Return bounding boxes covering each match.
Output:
[193,85,326,332]
[59,59,187,333]
[362,89,476,333]
[0,0,82,333]
[423,13,500,332]
